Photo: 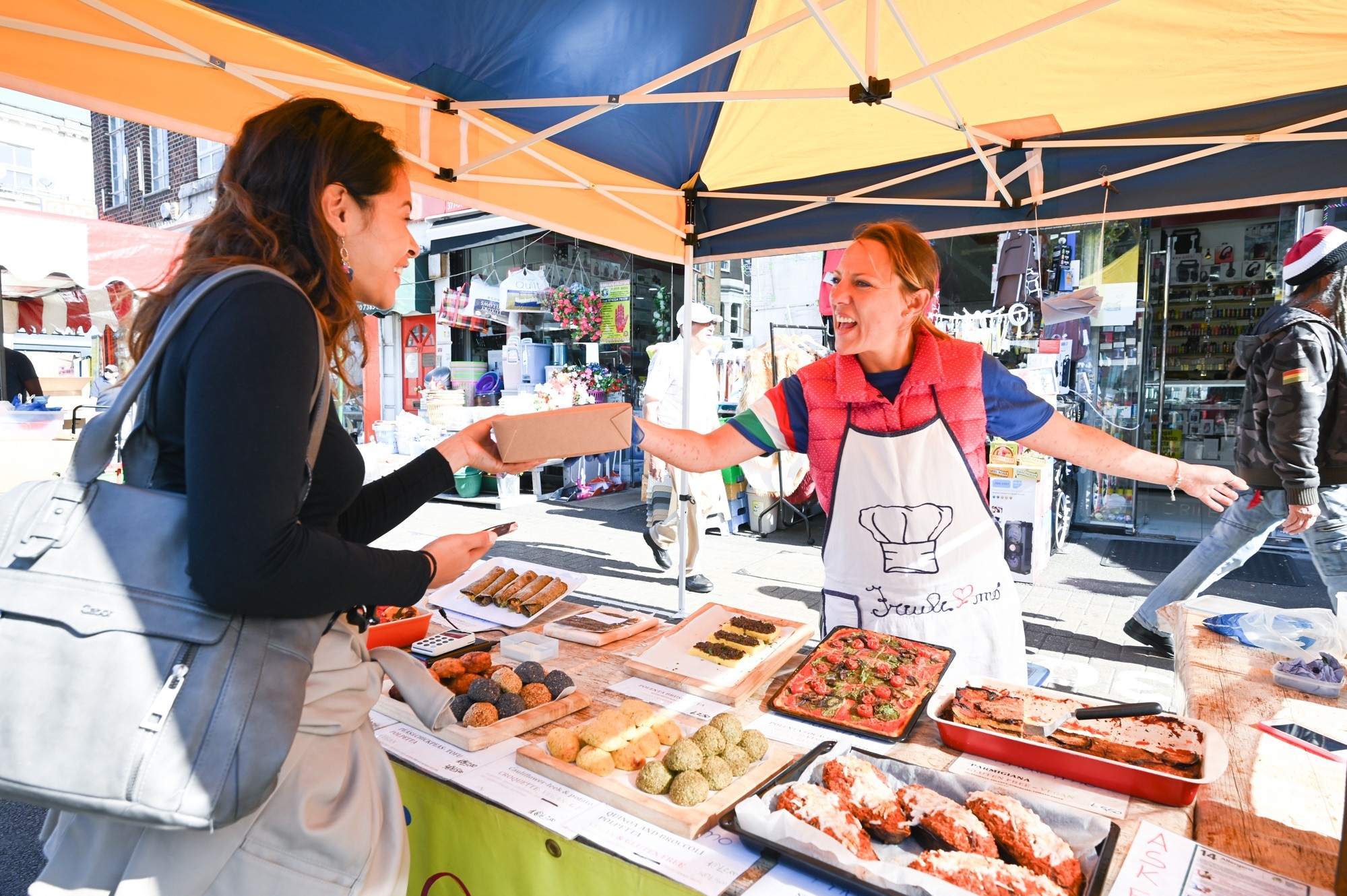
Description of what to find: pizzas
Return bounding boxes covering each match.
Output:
[772,628,949,737]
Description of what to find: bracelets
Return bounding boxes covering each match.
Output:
[1167,458,1181,502]
[416,550,437,586]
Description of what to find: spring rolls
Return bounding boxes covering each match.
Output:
[459,566,567,617]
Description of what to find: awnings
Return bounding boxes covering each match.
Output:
[0,203,191,334]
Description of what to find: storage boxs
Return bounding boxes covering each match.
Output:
[1271,661,1346,698]
[1159,245,1282,285]
[989,436,1052,586]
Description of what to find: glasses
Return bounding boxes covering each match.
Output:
[104,369,113,373]
[700,322,717,327]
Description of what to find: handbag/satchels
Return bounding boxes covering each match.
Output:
[437,281,487,333]
[498,267,553,313]
[461,270,509,326]
[0,263,329,833]
[1202,607,1347,666]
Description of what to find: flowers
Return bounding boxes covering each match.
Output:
[529,364,622,410]
[555,286,603,342]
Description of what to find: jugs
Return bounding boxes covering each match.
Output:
[593,261,619,280]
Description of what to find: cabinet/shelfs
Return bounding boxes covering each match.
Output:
[1082,304,1149,531]
[1146,279,1276,372]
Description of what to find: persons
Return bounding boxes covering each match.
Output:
[643,302,723,593]
[30,98,546,896]
[0,345,44,405]
[1123,224,1347,658]
[90,364,123,471]
[632,219,1250,685]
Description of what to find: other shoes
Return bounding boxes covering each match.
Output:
[1123,617,1175,659]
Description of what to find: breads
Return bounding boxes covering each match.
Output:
[950,686,1204,778]
[689,615,778,667]
[776,755,1082,896]
[388,654,768,809]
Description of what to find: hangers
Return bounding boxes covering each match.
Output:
[760,322,827,358]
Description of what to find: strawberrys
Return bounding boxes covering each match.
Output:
[553,288,604,341]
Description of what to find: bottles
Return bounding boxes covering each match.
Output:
[1146,282,1278,370]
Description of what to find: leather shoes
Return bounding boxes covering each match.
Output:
[677,575,713,592]
[643,528,672,570]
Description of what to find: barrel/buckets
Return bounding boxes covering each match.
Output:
[502,342,568,391]
[474,392,501,406]
[453,466,484,498]
[372,424,398,453]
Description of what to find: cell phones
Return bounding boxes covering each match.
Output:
[483,522,518,537]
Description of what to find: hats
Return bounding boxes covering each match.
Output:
[105,364,118,372]
[1282,226,1347,286]
[676,302,723,328]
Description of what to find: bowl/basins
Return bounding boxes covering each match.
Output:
[424,366,456,388]
[476,371,503,394]
[365,604,434,650]
[925,676,1230,807]
[638,376,647,382]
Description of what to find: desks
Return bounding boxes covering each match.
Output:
[369,586,1196,896]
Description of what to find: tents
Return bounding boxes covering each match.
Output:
[0,0,1347,611]
